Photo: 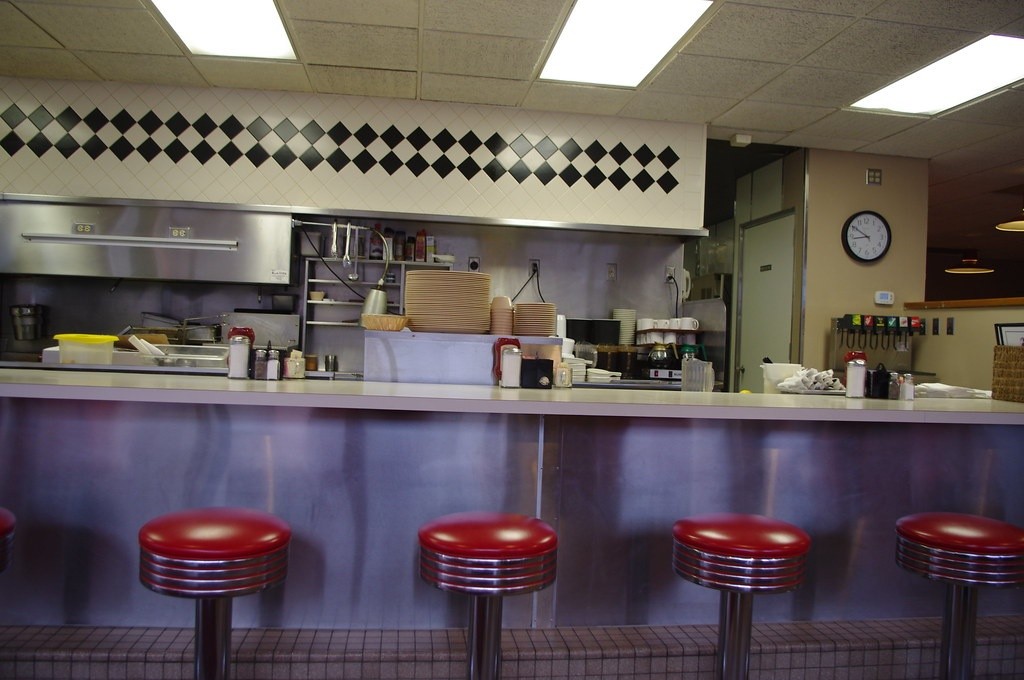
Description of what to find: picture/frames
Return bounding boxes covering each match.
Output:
[995,322,1024,346]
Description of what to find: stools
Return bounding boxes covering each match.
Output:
[672,513,812,680]
[418,512,557,680]
[894,512,1024,680]
[0,506,16,572]
[138,509,291,680]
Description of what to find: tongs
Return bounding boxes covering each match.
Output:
[343,222,352,269]
[330,221,338,257]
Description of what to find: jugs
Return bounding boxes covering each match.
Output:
[647,343,708,368]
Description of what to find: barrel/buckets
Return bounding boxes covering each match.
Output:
[10,305,42,340]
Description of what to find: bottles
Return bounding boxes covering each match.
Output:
[267,350,280,380]
[228,335,250,379]
[325,355,336,371]
[416,228,426,262]
[369,223,382,260]
[394,230,406,261]
[305,354,318,371]
[501,348,522,387]
[406,236,416,261]
[846,360,914,400]
[383,226,394,260]
[255,350,267,380]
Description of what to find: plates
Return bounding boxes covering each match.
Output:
[513,302,556,336]
[405,270,491,334]
[613,309,636,345]
[775,387,847,394]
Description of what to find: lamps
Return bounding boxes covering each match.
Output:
[945,247,995,275]
[995,186,1024,232]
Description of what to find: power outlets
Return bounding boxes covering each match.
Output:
[169,227,191,239]
[866,168,881,185]
[528,260,540,278]
[72,223,96,234]
[665,266,676,284]
[606,263,617,281]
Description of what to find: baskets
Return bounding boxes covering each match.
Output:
[362,313,409,331]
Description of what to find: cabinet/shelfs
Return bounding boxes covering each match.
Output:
[300,257,453,381]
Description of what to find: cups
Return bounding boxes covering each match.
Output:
[491,297,513,335]
[637,318,699,331]
[637,331,696,346]
[681,360,712,391]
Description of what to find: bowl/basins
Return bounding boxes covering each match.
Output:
[310,291,325,301]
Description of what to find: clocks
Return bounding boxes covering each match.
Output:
[840,210,892,263]
